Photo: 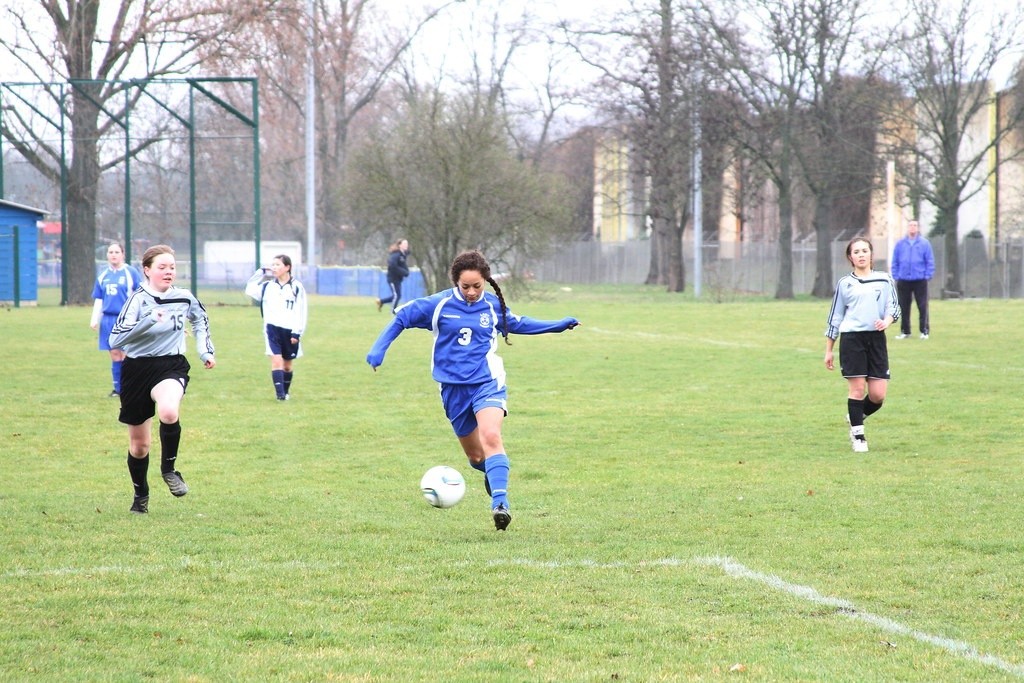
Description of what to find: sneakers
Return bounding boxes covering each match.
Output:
[853,440,868,452]
[485,474,491,496]
[493,502,512,530]
[846,413,857,442]
[129,494,149,514]
[161,469,187,497]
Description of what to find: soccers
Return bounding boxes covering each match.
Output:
[419,463,468,508]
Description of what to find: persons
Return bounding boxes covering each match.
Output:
[107,244,215,512]
[891,219,935,339]
[825,236,901,453]
[245,253,307,401]
[367,252,582,531]
[90,242,142,396]
[377,237,410,315]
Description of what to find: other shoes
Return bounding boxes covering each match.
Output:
[376,298,382,313]
[920,333,929,339]
[895,333,912,339]
[276,394,289,401]
